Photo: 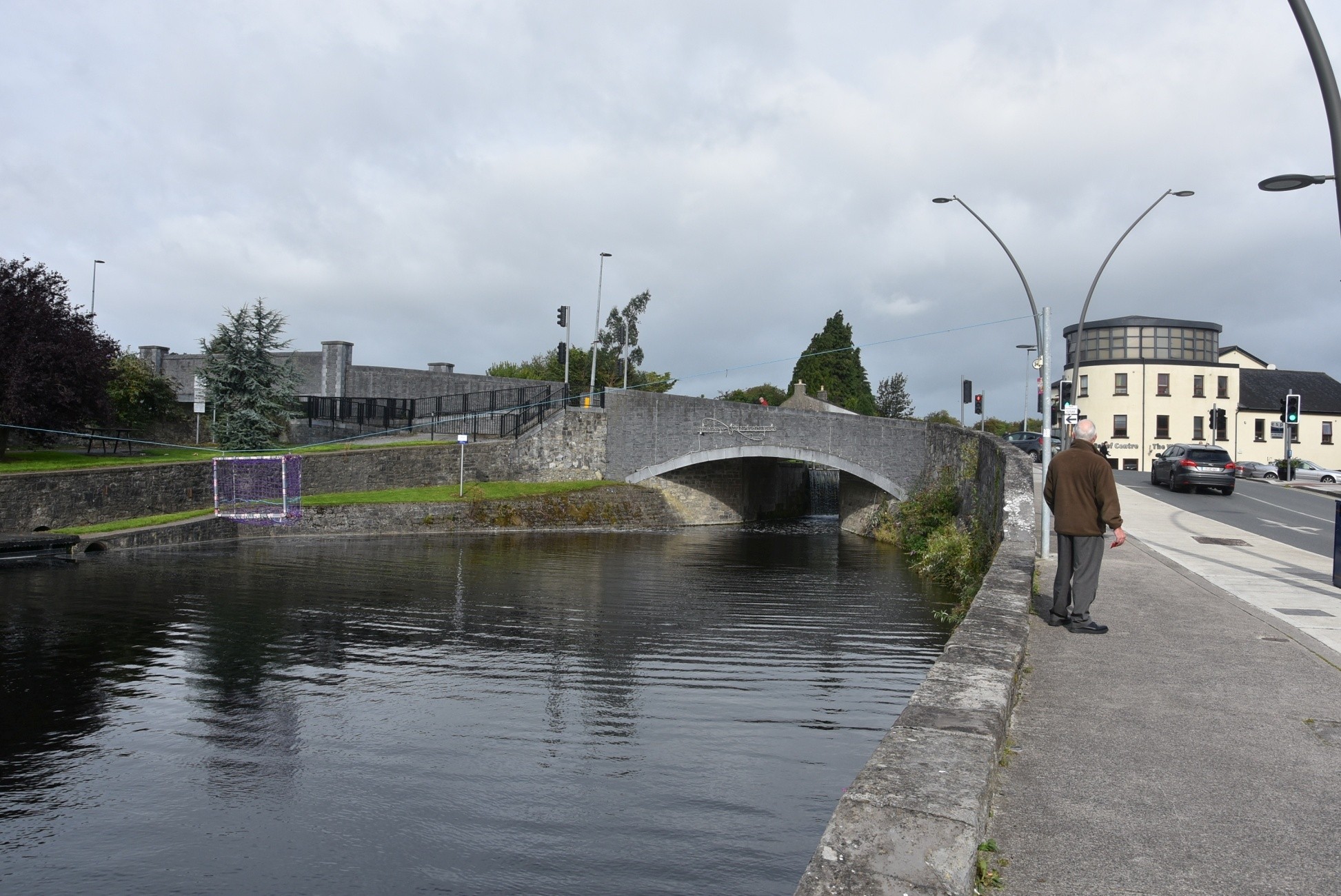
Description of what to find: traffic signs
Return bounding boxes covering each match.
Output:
[1065,405,1078,424]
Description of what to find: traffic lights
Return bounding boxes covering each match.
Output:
[975,394,983,415]
[1280,398,1286,423]
[557,306,566,327]
[1286,394,1301,424]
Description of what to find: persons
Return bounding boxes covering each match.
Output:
[758,396,768,406]
[1100,441,1111,458]
[1043,419,1126,634]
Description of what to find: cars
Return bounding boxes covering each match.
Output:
[1253,459,1341,484]
[1152,443,1236,496]
[1234,460,1262,479]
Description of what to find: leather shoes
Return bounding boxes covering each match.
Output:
[1048,619,1071,626]
[1069,622,1108,634]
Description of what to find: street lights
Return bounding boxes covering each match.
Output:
[1064,188,1194,449]
[932,195,1051,557]
[90,260,105,324]
[1024,349,1036,432]
[589,253,611,407]
